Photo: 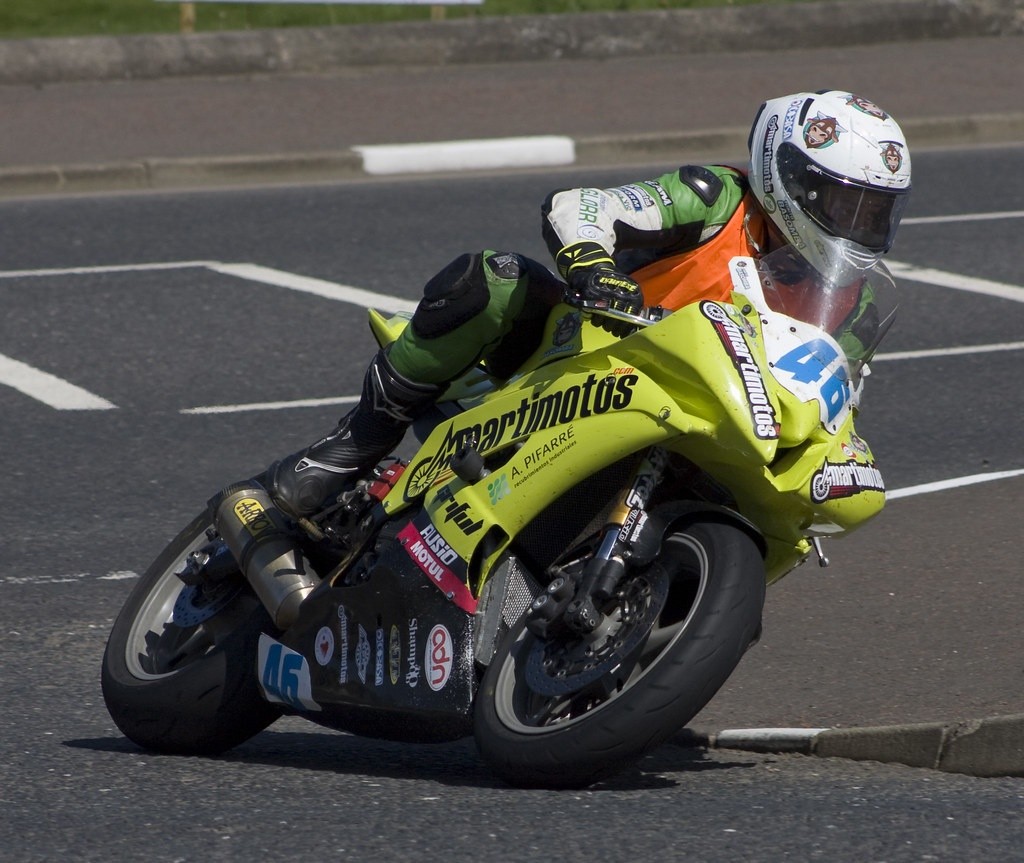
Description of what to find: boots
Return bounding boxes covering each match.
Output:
[266,337,450,520]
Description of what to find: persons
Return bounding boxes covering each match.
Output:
[266,91,913,518]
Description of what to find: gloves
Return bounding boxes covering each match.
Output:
[555,240,644,341]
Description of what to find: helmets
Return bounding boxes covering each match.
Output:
[748,92,911,283]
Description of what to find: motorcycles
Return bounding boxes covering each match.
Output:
[101,239,903,789]
